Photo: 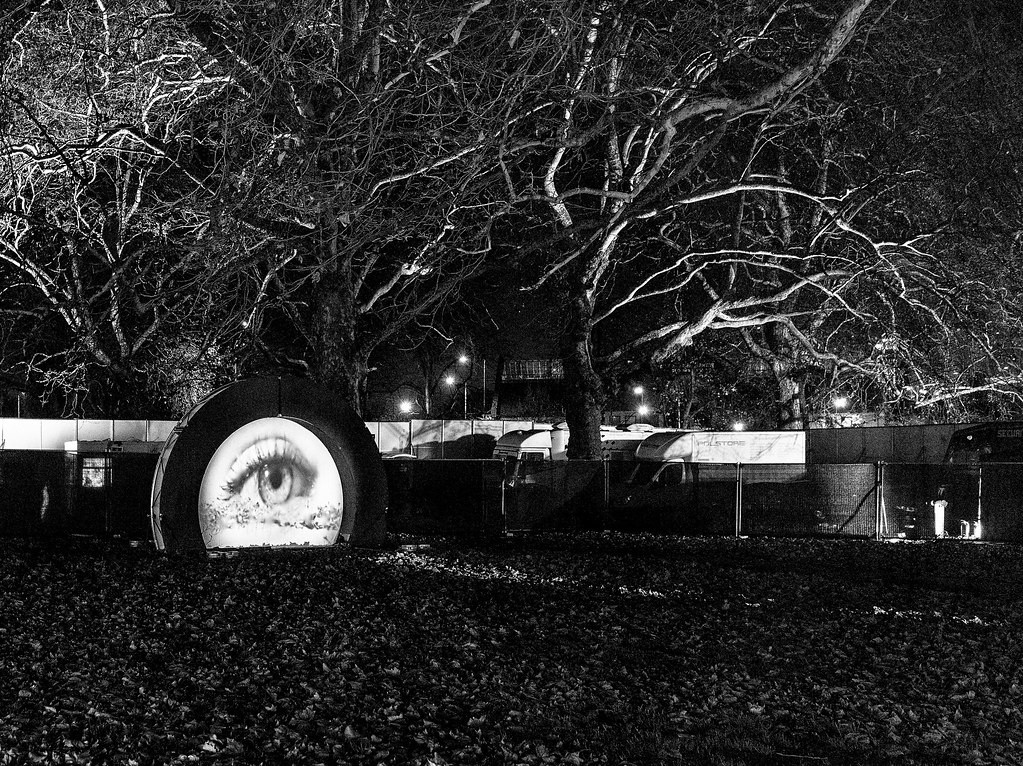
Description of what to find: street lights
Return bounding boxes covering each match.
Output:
[461,357,487,409]
[446,378,468,420]
[633,387,644,424]
[834,401,845,429]
[17,391,26,418]
